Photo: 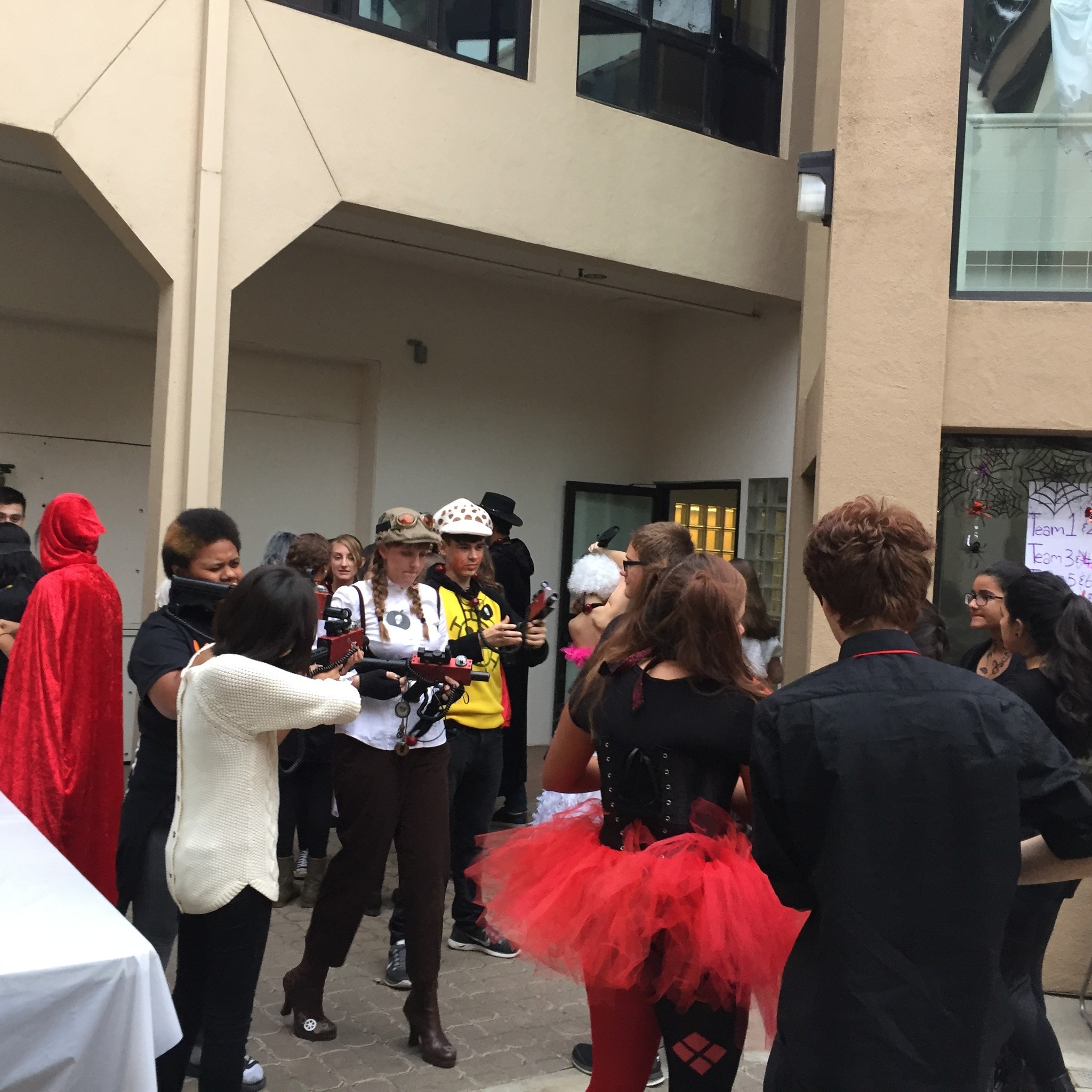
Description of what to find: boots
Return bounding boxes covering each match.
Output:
[302,857,327,909]
[267,855,303,909]
[279,958,339,1042]
[402,980,458,1068]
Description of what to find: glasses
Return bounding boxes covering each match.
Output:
[621,559,643,571]
[376,512,434,533]
[965,592,1004,606]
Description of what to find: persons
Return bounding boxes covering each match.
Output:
[992,564,1092,1092]
[150,566,366,1090]
[254,485,786,918]
[956,564,1031,1087]
[0,485,49,699]
[1,492,124,908]
[277,508,467,1070]
[746,496,1090,1092]
[463,548,815,1091]
[382,495,523,990]
[111,509,271,1089]
[902,595,950,665]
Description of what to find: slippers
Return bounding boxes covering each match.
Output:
[184,1045,266,1092]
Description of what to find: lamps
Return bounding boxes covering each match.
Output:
[794,145,836,229]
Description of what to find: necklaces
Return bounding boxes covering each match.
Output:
[979,639,1013,679]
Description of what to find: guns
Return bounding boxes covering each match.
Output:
[478,581,560,655]
[162,573,352,644]
[276,619,370,677]
[348,644,491,747]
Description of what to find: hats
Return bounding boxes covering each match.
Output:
[374,507,442,546]
[476,491,524,528]
[433,497,495,537]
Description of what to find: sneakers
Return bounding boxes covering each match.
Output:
[569,1040,667,1087]
[293,850,308,878]
[384,943,413,989]
[447,921,521,957]
